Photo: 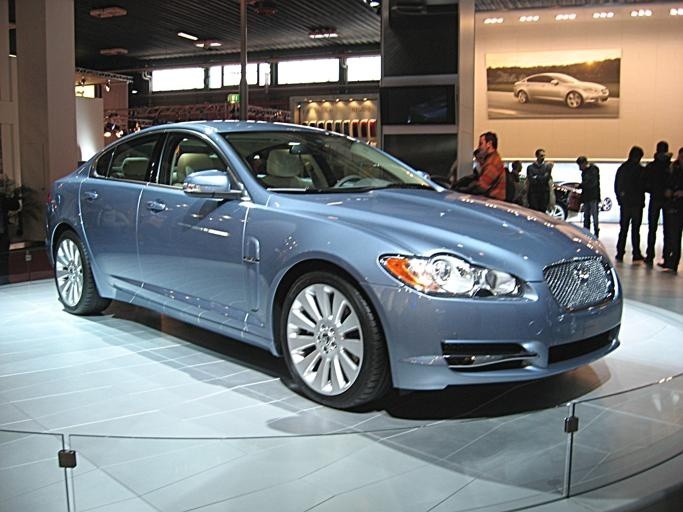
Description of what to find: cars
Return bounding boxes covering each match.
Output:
[44,119,623,409]
[533,181,612,222]
[513,72,609,109]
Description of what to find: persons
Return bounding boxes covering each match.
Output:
[576,156,601,239]
[467,132,556,213]
[614,140,683,273]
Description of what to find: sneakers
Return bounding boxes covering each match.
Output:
[615,251,678,273]
